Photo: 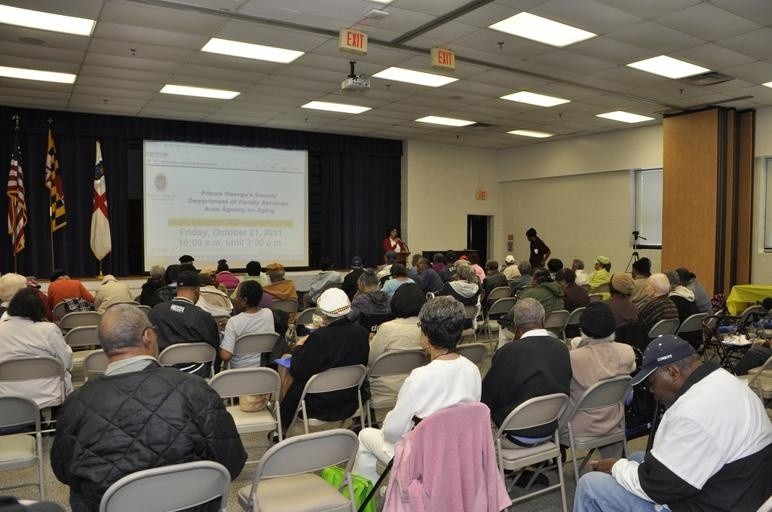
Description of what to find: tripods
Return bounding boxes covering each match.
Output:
[625,241,640,274]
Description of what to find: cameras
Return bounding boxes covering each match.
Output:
[632,231,647,240]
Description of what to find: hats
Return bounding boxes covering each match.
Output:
[102,274,118,284]
[177,270,203,286]
[592,255,611,265]
[612,272,635,296]
[351,256,365,269]
[505,255,514,263]
[628,334,697,386]
[180,255,194,263]
[267,263,284,273]
[580,301,617,338]
[633,258,652,277]
[317,287,351,317]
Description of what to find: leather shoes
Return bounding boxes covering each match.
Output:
[268,432,285,442]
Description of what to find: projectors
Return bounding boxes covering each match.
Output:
[341,77,371,93]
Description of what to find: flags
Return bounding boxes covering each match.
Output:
[7,119,28,273]
[90,139,111,274]
[45,128,67,271]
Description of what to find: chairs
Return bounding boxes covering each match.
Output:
[0,395,46,504]
[741,306,763,320]
[296,307,324,332]
[158,342,217,386]
[544,310,569,343]
[61,312,102,331]
[220,332,281,407]
[453,342,489,371]
[287,364,365,449]
[676,313,707,350]
[1,353,67,436]
[647,318,680,340]
[426,291,480,343]
[237,429,357,511]
[569,373,636,485]
[700,315,753,372]
[139,305,151,315]
[99,460,231,512]
[369,348,427,428]
[479,298,514,341]
[615,321,644,349]
[487,286,512,302]
[495,394,571,512]
[388,402,508,512]
[568,306,587,325]
[211,367,283,448]
[211,315,232,334]
[52,300,92,322]
[83,347,111,384]
[62,326,103,351]
[271,298,298,323]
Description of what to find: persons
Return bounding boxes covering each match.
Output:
[408,254,653,299]
[343,256,366,300]
[480,299,573,449]
[147,270,222,378]
[211,259,239,289]
[675,267,713,316]
[231,261,271,300]
[603,273,638,328]
[573,335,772,512]
[351,269,391,323]
[179,255,194,265]
[218,281,275,371]
[269,289,370,445]
[384,226,405,253]
[49,304,249,511]
[94,274,134,315]
[732,340,772,376]
[0,289,73,436]
[663,269,704,349]
[150,265,183,308]
[377,251,398,288]
[637,273,678,333]
[553,302,636,447]
[261,264,298,302]
[367,284,426,408]
[140,265,165,308]
[0,273,27,317]
[352,295,481,485]
[47,269,95,337]
[195,273,234,333]
[526,227,550,268]
[303,257,343,310]
[381,263,413,295]
